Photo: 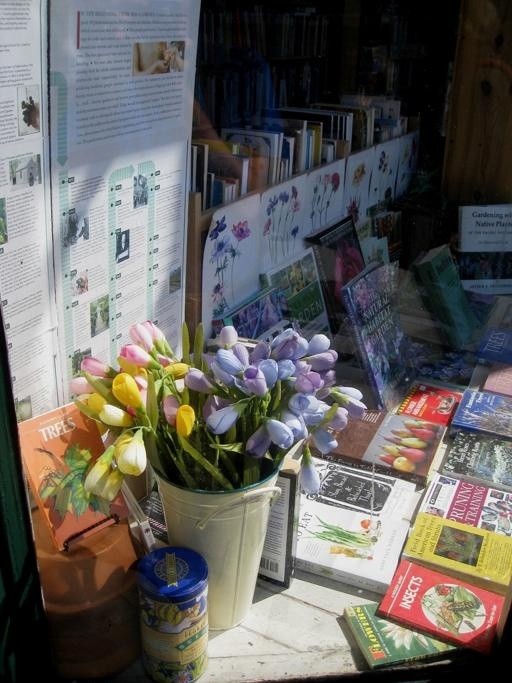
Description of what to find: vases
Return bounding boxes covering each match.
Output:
[154,470,279,632]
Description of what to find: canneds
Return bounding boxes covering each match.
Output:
[132,546,209,683]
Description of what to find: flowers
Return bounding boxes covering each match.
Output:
[39,324,368,518]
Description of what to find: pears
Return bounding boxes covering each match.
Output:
[376,419,439,473]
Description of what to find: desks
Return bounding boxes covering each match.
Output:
[111,570,450,683]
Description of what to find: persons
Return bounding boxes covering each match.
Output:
[117,232,129,262]
[135,42,183,73]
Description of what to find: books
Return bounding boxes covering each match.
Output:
[16,399,170,555]
[201,204,512,667]
[184,93,409,204]
